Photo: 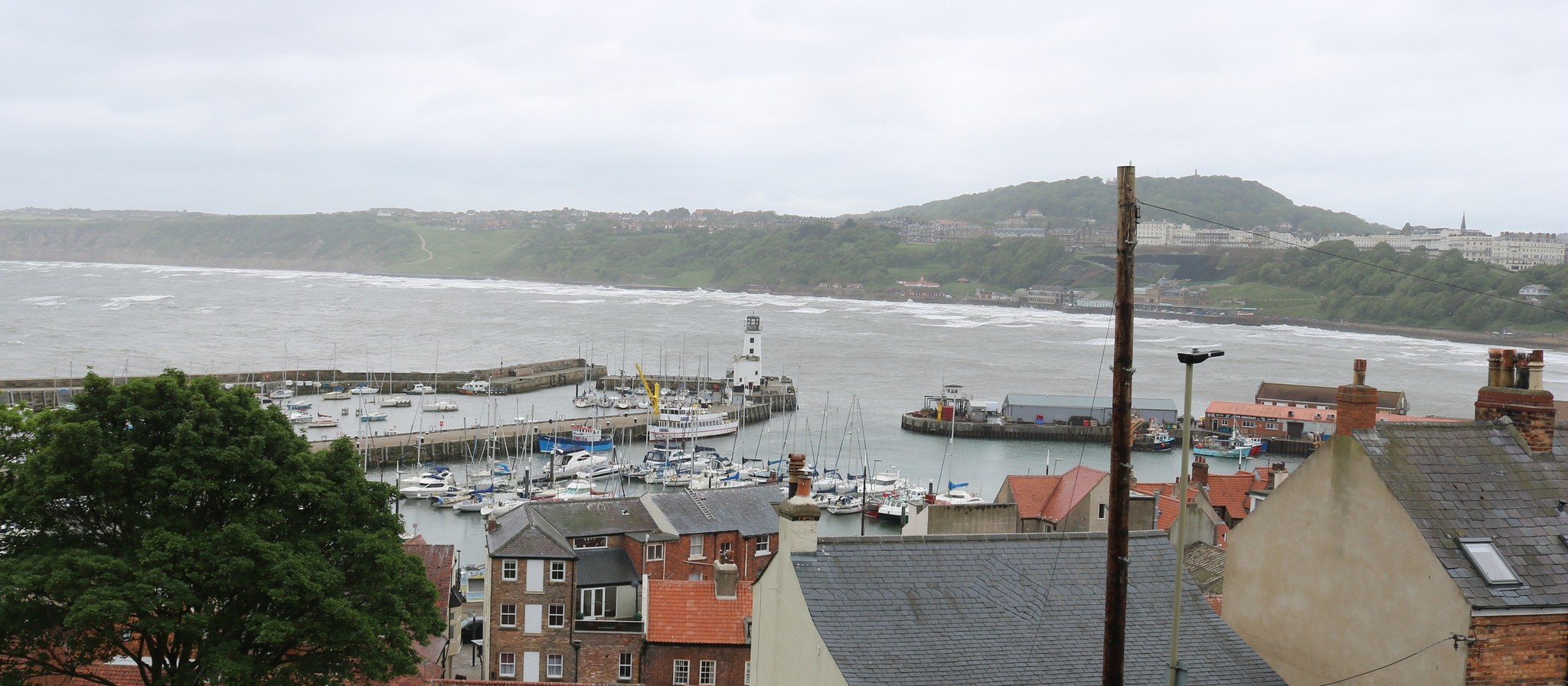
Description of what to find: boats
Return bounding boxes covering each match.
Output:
[1307,419,1336,451]
[1191,406,1270,459]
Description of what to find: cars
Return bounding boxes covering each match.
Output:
[461,616,483,645]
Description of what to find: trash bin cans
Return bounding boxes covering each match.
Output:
[549,420,552,424]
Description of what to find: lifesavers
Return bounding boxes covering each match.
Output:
[671,477,675,481]
[1226,442,1229,447]
[576,437,579,441]
[1221,442,1225,446]
[1199,440,1202,442]
[488,504,492,508]
[716,484,719,487]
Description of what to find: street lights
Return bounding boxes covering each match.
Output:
[1165,348,1227,686]
[457,550,460,592]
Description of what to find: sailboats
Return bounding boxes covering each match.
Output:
[0,328,987,561]
